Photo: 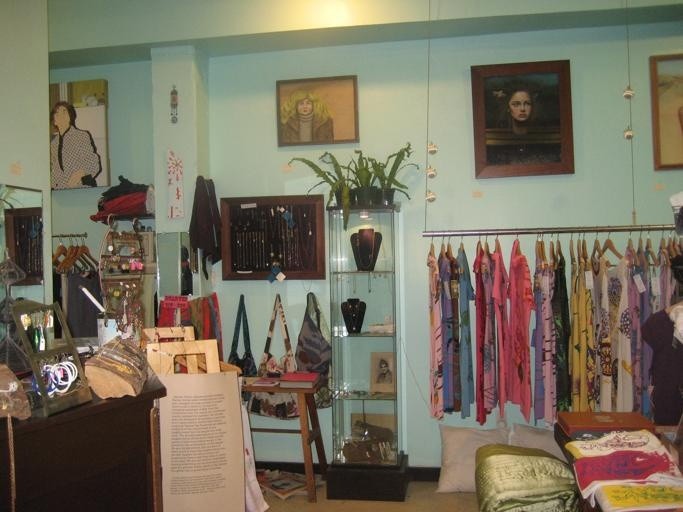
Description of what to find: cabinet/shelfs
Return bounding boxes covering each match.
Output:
[321,201,409,503]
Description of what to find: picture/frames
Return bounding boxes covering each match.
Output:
[646,52,683,173]
[273,75,362,148]
[469,58,578,180]
[366,348,398,399]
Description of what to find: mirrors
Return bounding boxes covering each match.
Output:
[0,184,44,380]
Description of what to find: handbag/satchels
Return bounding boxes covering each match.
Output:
[249,351,300,419]
[227,353,256,401]
[308,363,331,409]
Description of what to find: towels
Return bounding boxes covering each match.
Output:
[186,173,223,265]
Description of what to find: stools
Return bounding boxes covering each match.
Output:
[242,377,327,503]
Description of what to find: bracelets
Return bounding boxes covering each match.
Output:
[28,360,80,397]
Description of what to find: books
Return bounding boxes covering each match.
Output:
[253,467,327,501]
[250,377,281,387]
[278,372,321,389]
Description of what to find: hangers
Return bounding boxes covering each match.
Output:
[50,233,100,278]
[427,223,682,272]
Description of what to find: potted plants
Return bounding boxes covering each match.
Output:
[286,144,422,232]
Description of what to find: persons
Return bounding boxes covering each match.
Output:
[375,358,391,383]
[281,88,333,142]
[49,104,100,191]
[485,78,563,168]
[640,300,683,427]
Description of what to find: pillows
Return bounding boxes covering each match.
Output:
[433,421,571,495]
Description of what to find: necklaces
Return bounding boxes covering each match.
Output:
[13,215,41,278]
[345,301,361,331]
[355,233,375,269]
[232,206,315,273]
